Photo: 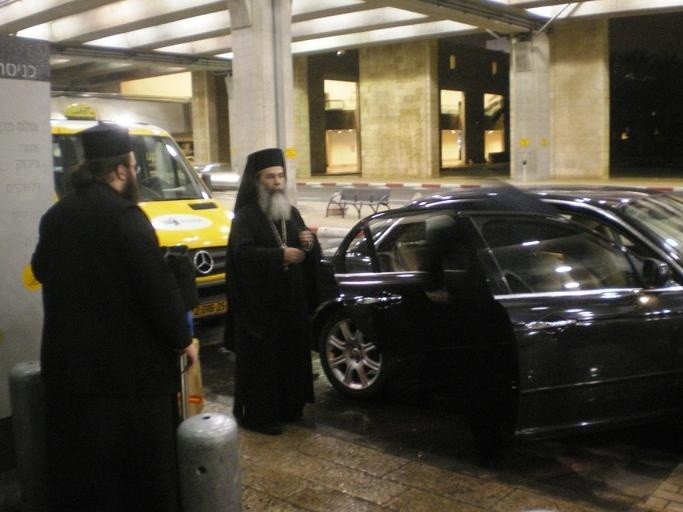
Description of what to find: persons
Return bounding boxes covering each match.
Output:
[220,148,339,436]
[28,123,198,512]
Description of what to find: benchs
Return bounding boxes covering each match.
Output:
[327,185,390,219]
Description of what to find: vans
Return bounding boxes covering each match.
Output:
[50,104,232,325]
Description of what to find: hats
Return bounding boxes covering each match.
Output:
[248,149,284,176]
[81,124,130,158]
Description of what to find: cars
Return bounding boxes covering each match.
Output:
[310,183,683,445]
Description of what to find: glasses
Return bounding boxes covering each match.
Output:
[126,164,141,173]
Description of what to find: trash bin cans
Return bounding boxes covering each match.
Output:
[10,361,47,488]
[175,412,244,512]
